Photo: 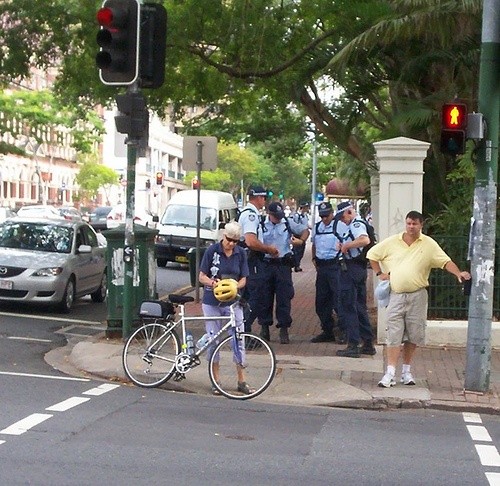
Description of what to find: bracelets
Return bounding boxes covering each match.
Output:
[376,271,382,276]
[300,238,304,242]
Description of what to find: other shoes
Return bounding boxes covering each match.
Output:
[279,330,289,343]
[260,328,270,342]
[212,381,223,395]
[311,332,335,342]
[245,338,262,348]
[238,381,255,394]
[336,349,360,358]
[359,346,376,355]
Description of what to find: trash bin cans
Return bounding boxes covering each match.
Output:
[101,223,161,338]
[185,246,209,288]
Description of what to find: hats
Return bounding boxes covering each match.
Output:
[318,202,332,216]
[334,202,352,217]
[268,202,284,219]
[300,202,308,209]
[251,186,267,197]
[375,280,390,307]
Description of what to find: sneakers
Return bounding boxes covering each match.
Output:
[376,372,396,388]
[400,371,415,385]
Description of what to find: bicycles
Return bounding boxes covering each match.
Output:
[123,294,277,401]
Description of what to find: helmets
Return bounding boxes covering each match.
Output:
[213,279,237,302]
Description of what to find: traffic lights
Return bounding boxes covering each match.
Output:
[96,0,137,84]
[268,191,273,198]
[193,178,199,189]
[146,179,151,189]
[114,93,144,138]
[278,194,283,199]
[443,103,466,131]
[157,172,162,184]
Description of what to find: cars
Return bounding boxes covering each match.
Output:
[0,206,113,228]
[0,217,110,316]
[106,204,157,229]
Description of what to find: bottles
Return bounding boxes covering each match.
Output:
[339,257,348,272]
[195,331,214,350]
[187,331,194,355]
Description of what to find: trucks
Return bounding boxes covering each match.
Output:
[155,189,239,268]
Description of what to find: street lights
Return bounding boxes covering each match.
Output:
[195,139,201,303]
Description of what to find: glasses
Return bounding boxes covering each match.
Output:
[225,236,239,243]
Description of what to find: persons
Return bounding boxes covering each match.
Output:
[237,186,279,349]
[310,202,348,345]
[366,211,471,388]
[199,221,257,396]
[259,202,309,345]
[335,202,377,358]
